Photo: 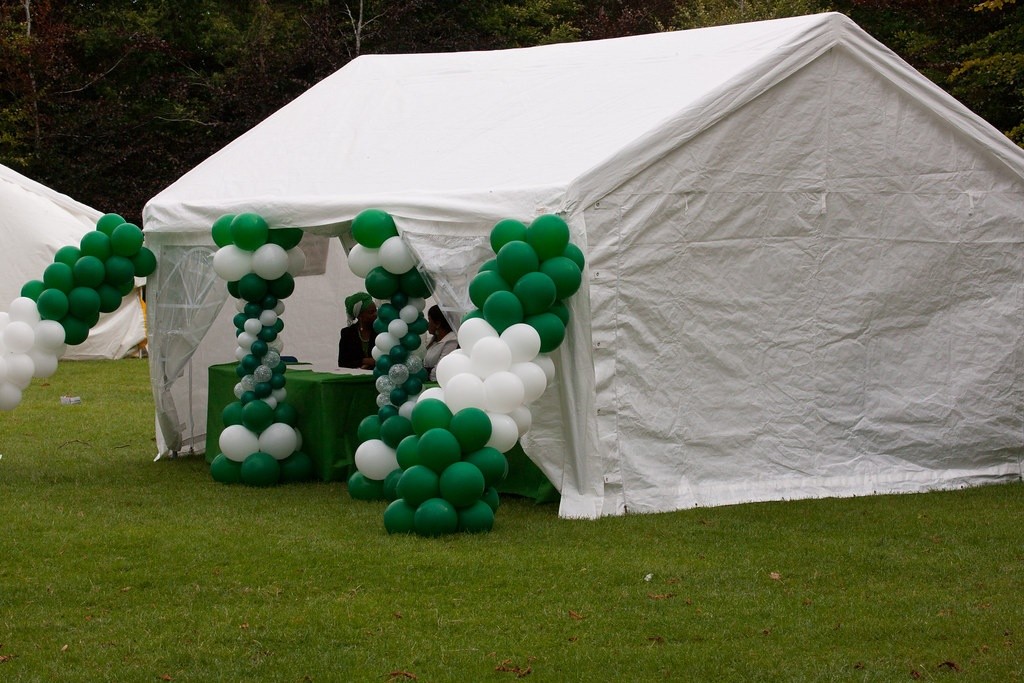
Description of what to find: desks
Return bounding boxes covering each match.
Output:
[204,356,376,484]
[408,383,553,504]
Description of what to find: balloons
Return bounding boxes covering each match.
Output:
[348,205,589,540]
[0,210,157,419]
[210,214,313,489]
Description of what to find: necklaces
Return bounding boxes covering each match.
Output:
[358,328,369,342]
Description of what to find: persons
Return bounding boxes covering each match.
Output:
[422,304,459,383]
[337,291,379,370]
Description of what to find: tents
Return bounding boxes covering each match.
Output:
[136,13,1024,522]
[0,157,152,360]
